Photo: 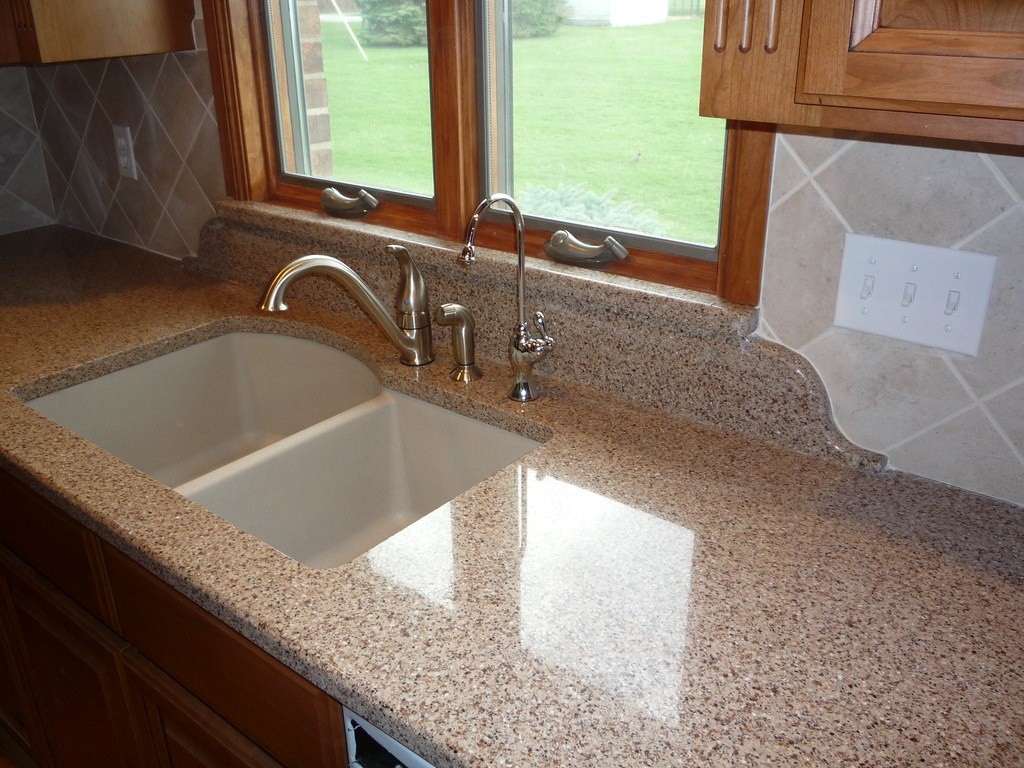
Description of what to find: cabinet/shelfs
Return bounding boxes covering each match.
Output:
[699,0,1024,150]
[0,0,196,65]
[0,469,349,768]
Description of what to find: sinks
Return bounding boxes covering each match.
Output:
[11,314,388,490]
[182,393,553,568]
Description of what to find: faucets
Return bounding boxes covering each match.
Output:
[259,244,434,367]
[460,192,558,401]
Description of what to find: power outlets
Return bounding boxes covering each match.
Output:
[112,125,138,180]
